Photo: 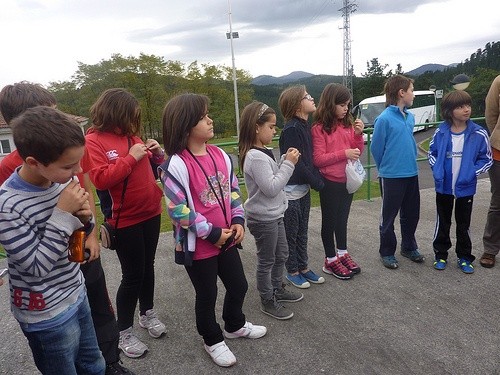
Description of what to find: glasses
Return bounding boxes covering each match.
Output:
[300,94,311,101]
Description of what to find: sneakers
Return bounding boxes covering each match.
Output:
[336,252,361,274]
[138,308,167,339]
[118,326,148,358]
[322,257,353,279]
[204,341,236,367]
[224,321,267,339]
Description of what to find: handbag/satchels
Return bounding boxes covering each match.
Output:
[100,222,116,251]
[345,157,366,194]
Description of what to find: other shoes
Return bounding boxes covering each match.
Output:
[259,298,294,320]
[106,363,135,375]
[480,253,495,268]
[276,287,303,303]
[433,259,447,270]
[400,250,426,263]
[285,271,310,289]
[456,258,475,274]
[300,270,325,283]
[381,255,399,268]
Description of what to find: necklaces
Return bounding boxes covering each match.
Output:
[189,146,230,228]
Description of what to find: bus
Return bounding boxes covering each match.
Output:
[351,89,437,145]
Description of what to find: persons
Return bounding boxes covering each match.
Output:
[156,94,266,366]
[427,89,494,273]
[237,100,304,320]
[369,74,426,269]
[0,81,135,374]
[276,84,329,289]
[84,88,168,358]
[0,105,107,374]
[480,74,500,267]
[311,82,365,280]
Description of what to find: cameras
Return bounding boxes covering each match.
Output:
[68,228,85,262]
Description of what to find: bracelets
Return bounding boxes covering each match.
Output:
[153,148,165,158]
